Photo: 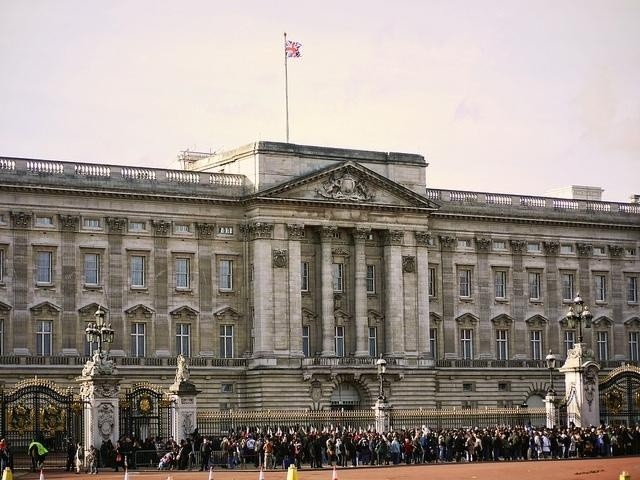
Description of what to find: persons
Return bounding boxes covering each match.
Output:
[2,419,640,480]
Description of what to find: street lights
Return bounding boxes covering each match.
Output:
[376,353,387,399]
[85,307,114,354]
[564,293,593,343]
[544,349,559,396]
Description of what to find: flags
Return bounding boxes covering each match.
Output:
[286,39,302,60]
